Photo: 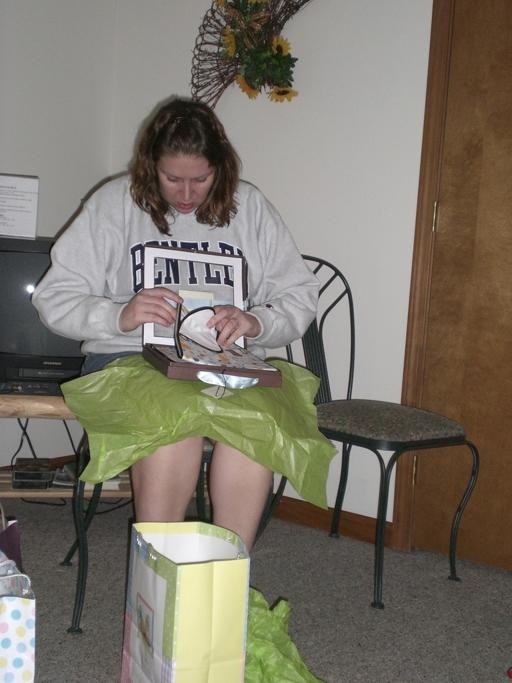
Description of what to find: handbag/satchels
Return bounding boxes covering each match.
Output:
[0,504,23,570]
[0,551,37,683]
[120,521,252,681]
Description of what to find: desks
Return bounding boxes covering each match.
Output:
[0,393,210,502]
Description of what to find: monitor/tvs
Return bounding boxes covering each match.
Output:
[0,234,88,396]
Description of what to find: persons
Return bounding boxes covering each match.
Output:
[30,98,323,552]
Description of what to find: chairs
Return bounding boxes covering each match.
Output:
[252,254,480,610]
[58,431,215,637]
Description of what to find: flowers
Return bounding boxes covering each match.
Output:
[213,1,299,104]
